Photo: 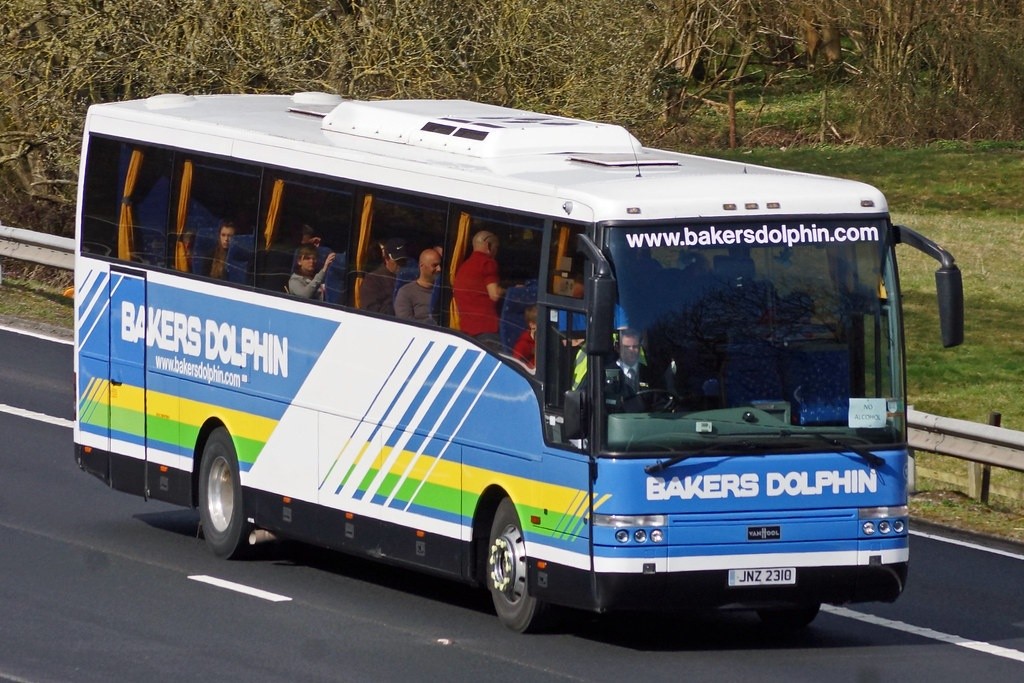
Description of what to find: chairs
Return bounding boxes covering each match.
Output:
[190,228,219,275]
[392,268,419,301]
[429,274,442,321]
[135,202,166,261]
[257,249,291,292]
[500,286,528,355]
[797,343,851,425]
[325,254,346,305]
[779,323,841,409]
[225,235,254,284]
[718,333,786,408]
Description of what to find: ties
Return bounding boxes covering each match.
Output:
[628,369,640,392]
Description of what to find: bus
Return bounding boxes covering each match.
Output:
[72,90,965,629]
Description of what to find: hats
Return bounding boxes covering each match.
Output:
[385,237,410,260]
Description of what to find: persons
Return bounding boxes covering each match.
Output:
[393,244,443,322]
[767,292,833,341]
[453,231,524,345]
[201,221,239,281]
[512,305,538,369]
[288,224,336,301]
[672,251,733,305]
[558,303,628,331]
[747,280,782,324]
[358,239,409,316]
[576,329,668,414]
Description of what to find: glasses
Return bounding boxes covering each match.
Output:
[299,255,320,261]
